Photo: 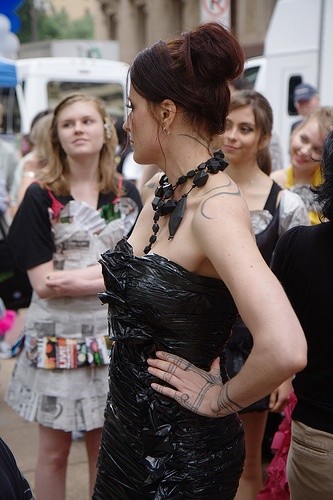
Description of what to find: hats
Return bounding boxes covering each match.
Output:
[293,84,318,100]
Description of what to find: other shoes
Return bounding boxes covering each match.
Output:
[0,333,24,359]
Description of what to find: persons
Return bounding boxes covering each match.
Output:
[211,78,333,500]
[7,93,143,500]
[92,21,307,500]
[0,95,163,440]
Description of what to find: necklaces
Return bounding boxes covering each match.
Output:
[143,149,229,254]
